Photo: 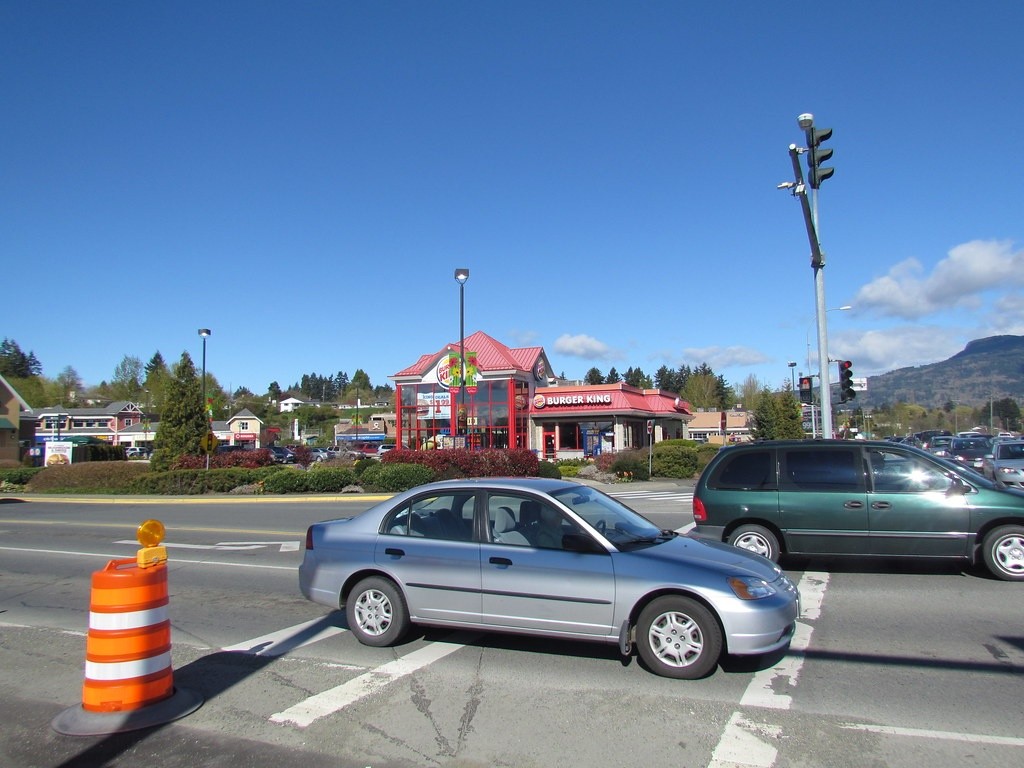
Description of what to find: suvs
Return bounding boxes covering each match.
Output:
[687,436,1024,581]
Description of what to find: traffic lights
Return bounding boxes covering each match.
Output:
[799,378,811,391]
[838,360,856,401]
[806,126,834,189]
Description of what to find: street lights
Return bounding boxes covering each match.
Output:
[197,328,211,429]
[805,305,851,440]
[454,268,470,405]
[788,361,797,400]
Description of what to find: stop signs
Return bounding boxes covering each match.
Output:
[720,411,726,430]
[647,419,653,434]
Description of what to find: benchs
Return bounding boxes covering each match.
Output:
[390,509,459,540]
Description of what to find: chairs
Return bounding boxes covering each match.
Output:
[492,507,529,546]
[519,501,539,538]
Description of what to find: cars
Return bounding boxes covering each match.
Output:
[125,447,152,460]
[215,442,410,464]
[298,475,802,681]
[868,428,1024,493]
